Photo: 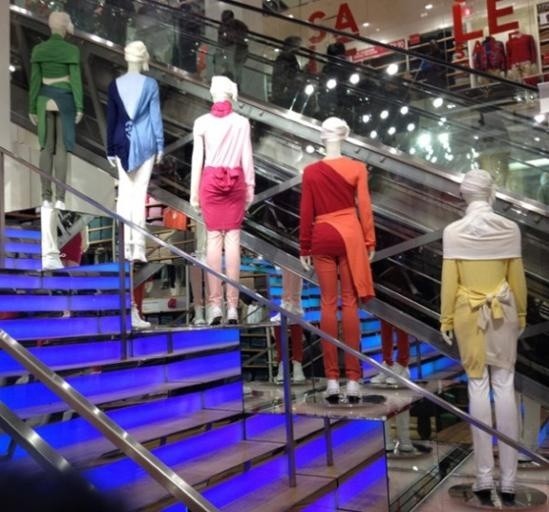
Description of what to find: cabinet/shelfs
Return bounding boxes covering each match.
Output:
[355,0,549,100]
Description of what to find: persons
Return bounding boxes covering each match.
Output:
[66,0,348,124]
[56,210,89,267]
[421,39,455,87]
[107,41,165,263]
[190,75,256,326]
[440,169,528,503]
[270,268,304,322]
[28,9,85,211]
[300,117,376,403]
[273,325,306,381]
[384,409,413,452]
[185,263,206,325]
[483,32,537,77]
[369,319,411,384]
